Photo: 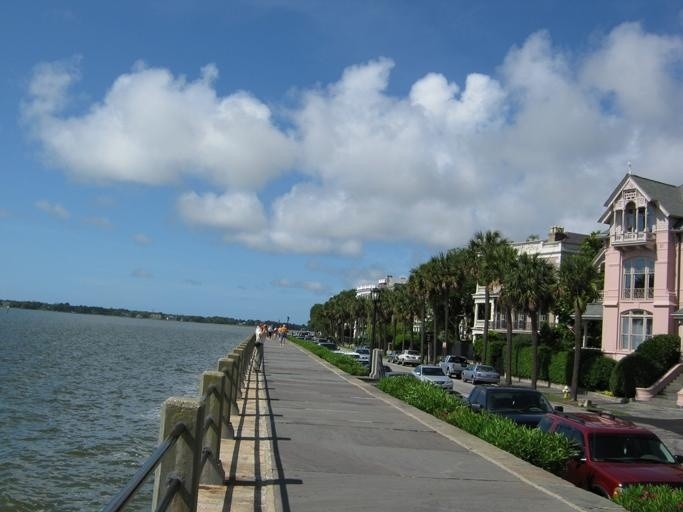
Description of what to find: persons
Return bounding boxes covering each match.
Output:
[251,322,288,373]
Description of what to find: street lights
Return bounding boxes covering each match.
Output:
[369,288,381,376]
